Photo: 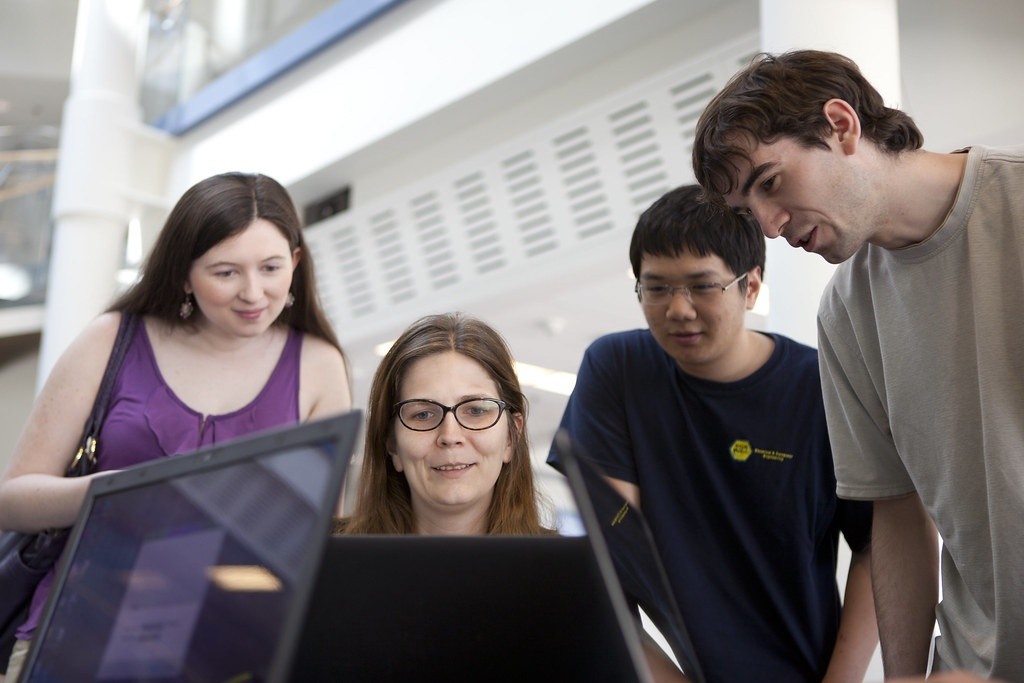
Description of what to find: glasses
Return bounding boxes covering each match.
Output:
[634,271,752,305]
[392,396,509,431]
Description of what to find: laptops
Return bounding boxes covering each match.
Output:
[16,407,648,683]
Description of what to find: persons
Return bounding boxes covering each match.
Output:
[692,50,1024,683]
[545,185,882,683]
[0,172,350,683]
[326,315,565,538]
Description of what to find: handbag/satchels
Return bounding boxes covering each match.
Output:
[0,311,139,678]
